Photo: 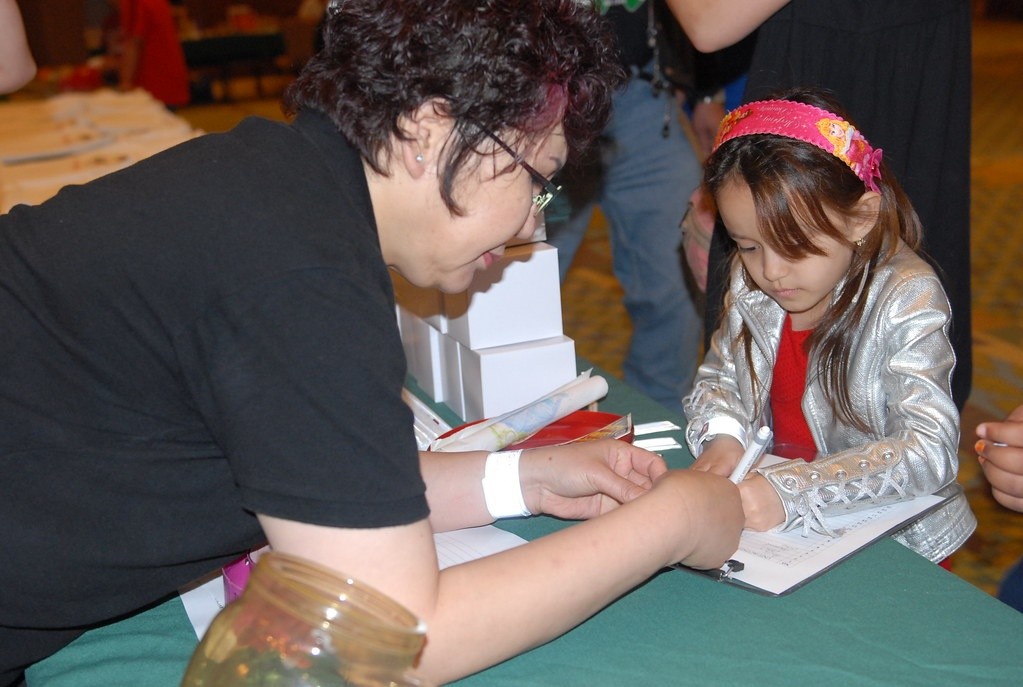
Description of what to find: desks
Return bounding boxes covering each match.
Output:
[22,354,1020,684]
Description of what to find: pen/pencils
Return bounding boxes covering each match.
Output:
[729,425,775,487]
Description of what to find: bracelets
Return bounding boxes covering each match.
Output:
[481,447,529,518]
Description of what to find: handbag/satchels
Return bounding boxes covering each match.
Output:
[680,183,716,295]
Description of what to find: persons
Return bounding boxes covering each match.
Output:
[0,0,744,687]
[971,410,1023,612]
[490,0,972,415]
[671,72,980,574]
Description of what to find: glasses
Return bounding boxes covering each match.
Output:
[459,114,561,217]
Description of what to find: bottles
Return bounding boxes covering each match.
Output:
[178,548,429,687]
